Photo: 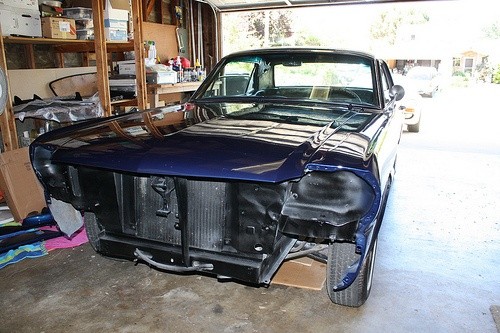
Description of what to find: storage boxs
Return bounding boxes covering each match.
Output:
[103,9,129,42]
[41,7,95,40]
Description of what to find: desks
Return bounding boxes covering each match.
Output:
[147,81,223,109]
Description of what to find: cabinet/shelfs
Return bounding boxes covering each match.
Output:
[0,0,163,152]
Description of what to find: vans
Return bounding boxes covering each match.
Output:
[407,66,439,98]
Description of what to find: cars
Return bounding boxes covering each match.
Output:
[392,72,423,132]
[28,45,407,309]
